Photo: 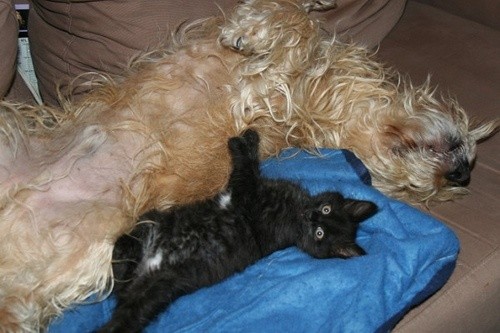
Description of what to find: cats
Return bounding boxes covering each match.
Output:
[85,127,377,333]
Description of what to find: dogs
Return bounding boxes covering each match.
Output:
[1,0,499,333]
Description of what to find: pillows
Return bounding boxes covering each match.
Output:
[42,144,460,333]
[25,0,407,108]
